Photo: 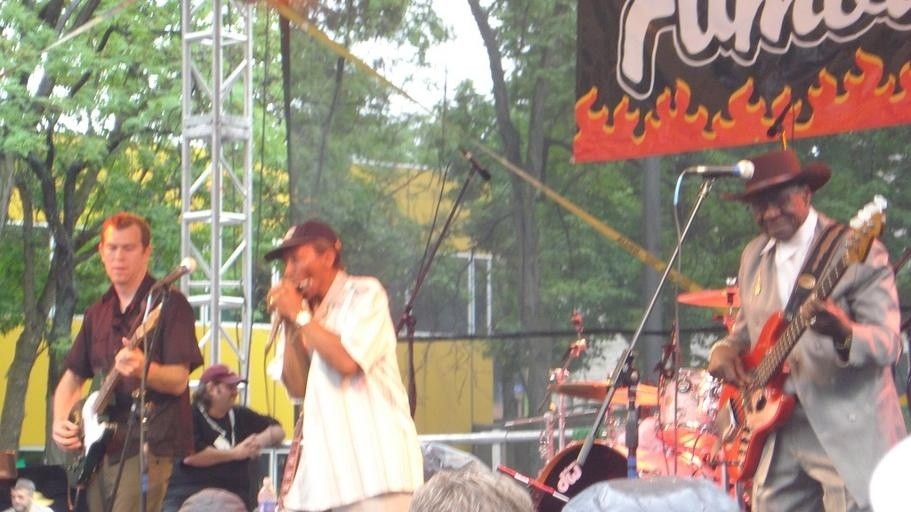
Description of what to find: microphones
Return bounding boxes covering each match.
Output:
[459,143,491,181]
[684,159,755,183]
[264,304,284,353]
[150,256,197,294]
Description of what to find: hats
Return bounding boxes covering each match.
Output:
[722,149,830,204]
[264,221,337,260]
[180,488,248,512]
[200,364,247,386]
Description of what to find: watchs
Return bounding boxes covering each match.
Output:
[296,309,313,327]
[833,332,853,352]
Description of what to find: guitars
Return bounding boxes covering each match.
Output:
[63,312,159,488]
[715,196,886,479]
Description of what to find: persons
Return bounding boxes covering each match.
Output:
[52,214,204,512]
[162,364,286,511]
[4,477,52,512]
[703,146,908,511]
[409,469,533,512]
[264,220,424,512]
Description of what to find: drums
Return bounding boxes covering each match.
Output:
[530,436,707,512]
[654,365,724,463]
[612,413,716,480]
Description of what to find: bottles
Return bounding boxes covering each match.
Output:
[257,476,278,511]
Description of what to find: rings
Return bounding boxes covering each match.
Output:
[269,295,273,304]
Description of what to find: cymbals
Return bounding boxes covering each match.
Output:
[556,379,659,406]
[675,288,740,308]
